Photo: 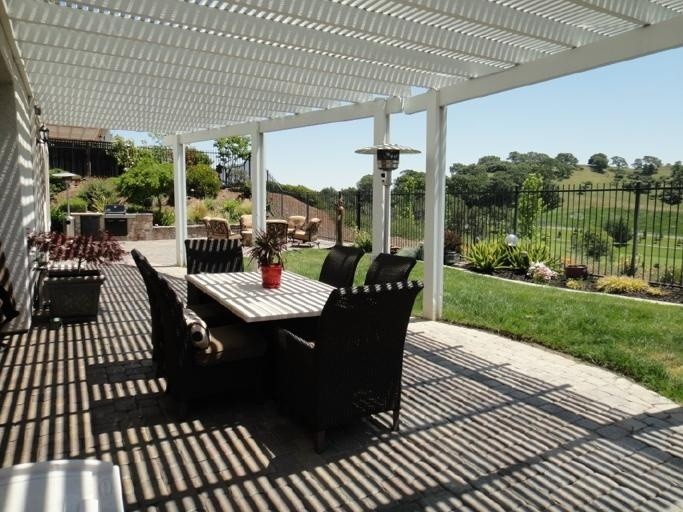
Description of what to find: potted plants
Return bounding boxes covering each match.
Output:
[445,229,464,264]
[250,225,288,289]
[24,228,130,320]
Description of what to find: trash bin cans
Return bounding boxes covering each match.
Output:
[81,215,100,237]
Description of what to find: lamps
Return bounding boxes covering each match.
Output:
[51,173,81,215]
[35,121,49,144]
[48,137,56,153]
[354,142,422,255]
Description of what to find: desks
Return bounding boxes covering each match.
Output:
[184,268,336,407]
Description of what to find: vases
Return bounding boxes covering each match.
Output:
[564,264,589,280]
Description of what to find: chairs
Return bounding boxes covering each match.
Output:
[128,248,243,416]
[262,279,425,454]
[201,212,321,247]
[318,244,362,291]
[363,254,417,286]
[184,237,243,305]
